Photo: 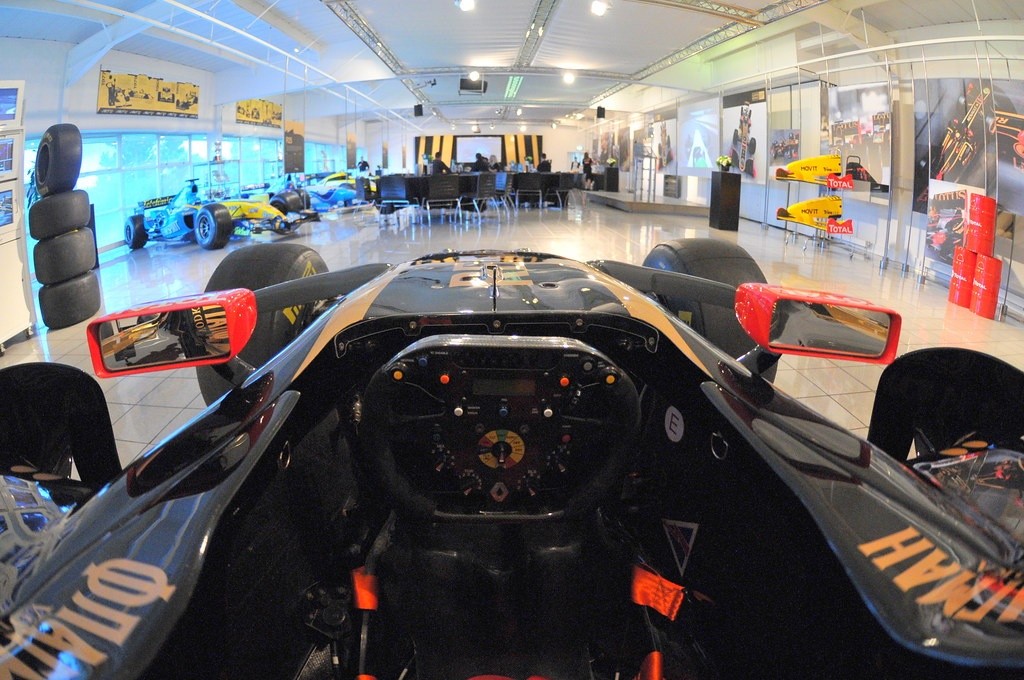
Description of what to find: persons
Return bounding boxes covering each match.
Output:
[357,151,551,190]
[570,157,580,171]
[582,153,594,190]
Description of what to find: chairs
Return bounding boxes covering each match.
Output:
[486,171,510,223]
[420,175,463,228]
[352,176,384,222]
[561,173,586,208]
[454,172,501,225]
[376,174,420,228]
[516,172,543,210]
[540,173,563,210]
[554,173,577,208]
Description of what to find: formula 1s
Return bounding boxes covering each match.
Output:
[124,178,313,251]
[281,168,381,215]
[726,100,757,179]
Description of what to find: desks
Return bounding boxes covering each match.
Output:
[378,173,484,224]
[505,171,570,208]
